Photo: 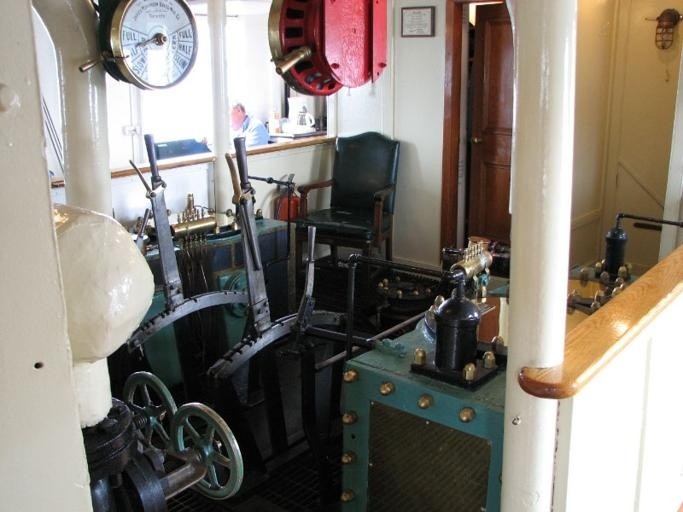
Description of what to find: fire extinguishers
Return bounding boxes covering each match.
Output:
[275,174,301,221]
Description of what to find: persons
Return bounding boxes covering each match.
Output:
[229,103,268,148]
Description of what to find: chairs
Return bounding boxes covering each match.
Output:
[292,132,400,274]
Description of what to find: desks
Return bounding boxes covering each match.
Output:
[50,119,339,273]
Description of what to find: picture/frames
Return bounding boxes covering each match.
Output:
[400,7,437,38]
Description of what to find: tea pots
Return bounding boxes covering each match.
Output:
[296,104,315,129]
